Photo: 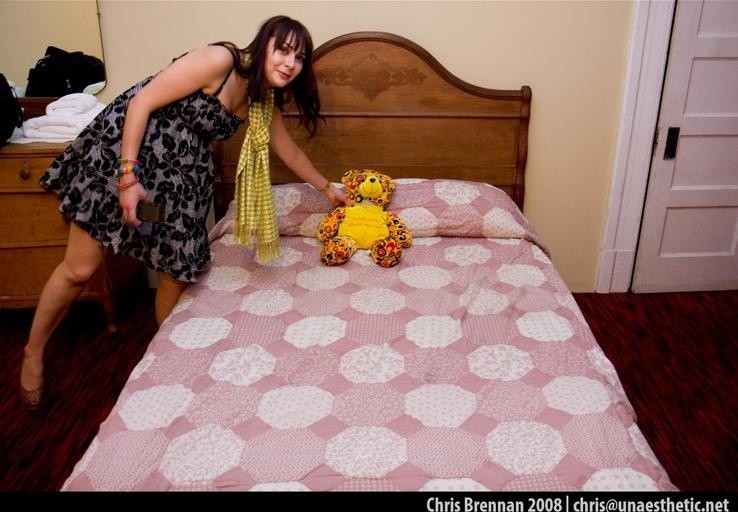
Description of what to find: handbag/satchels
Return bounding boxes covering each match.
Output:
[27,45,107,98]
[1,74,25,147]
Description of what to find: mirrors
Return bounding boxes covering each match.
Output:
[0,0,107,99]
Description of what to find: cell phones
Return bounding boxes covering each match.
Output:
[137,201,165,224]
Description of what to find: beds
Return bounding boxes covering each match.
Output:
[59,31,682,493]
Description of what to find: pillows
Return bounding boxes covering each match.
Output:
[207,177,553,261]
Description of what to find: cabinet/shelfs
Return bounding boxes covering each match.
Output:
[0,142,143,334]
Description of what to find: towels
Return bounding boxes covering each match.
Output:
[22,93,106,139]
[83,80,106,95]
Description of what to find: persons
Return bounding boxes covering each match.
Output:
[14,14,356,414]
[317,166,413,267]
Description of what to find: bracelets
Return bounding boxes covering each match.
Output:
[115,157,142,165]
[110,166,142,174]
[114,177,140,189]
[316,180,332,194]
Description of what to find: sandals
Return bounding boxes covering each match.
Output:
[19,347,47,409]
